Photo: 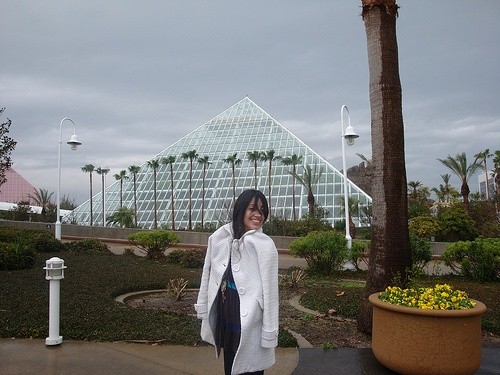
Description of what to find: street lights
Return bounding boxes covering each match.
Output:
[341,105,359,246]
[54,117,83,242]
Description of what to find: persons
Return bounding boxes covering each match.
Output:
[194,189,279,375]
